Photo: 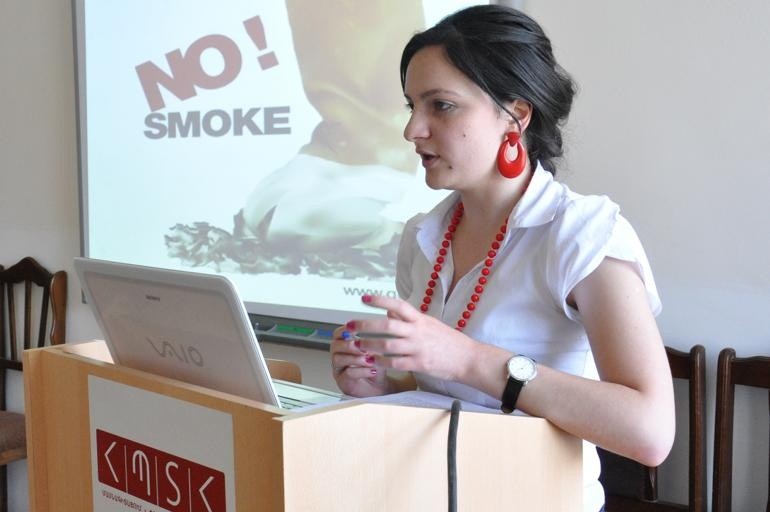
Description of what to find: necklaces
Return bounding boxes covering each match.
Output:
[422,201,510,332]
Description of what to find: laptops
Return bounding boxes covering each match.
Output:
[71,256,358,410]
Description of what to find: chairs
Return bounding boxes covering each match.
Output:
[0,257,67,512]
[605,345,707,512]
[712,348,770,512]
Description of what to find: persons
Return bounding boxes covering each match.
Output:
[329,5,675,509]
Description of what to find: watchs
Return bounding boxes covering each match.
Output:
[496,351,545,411]
